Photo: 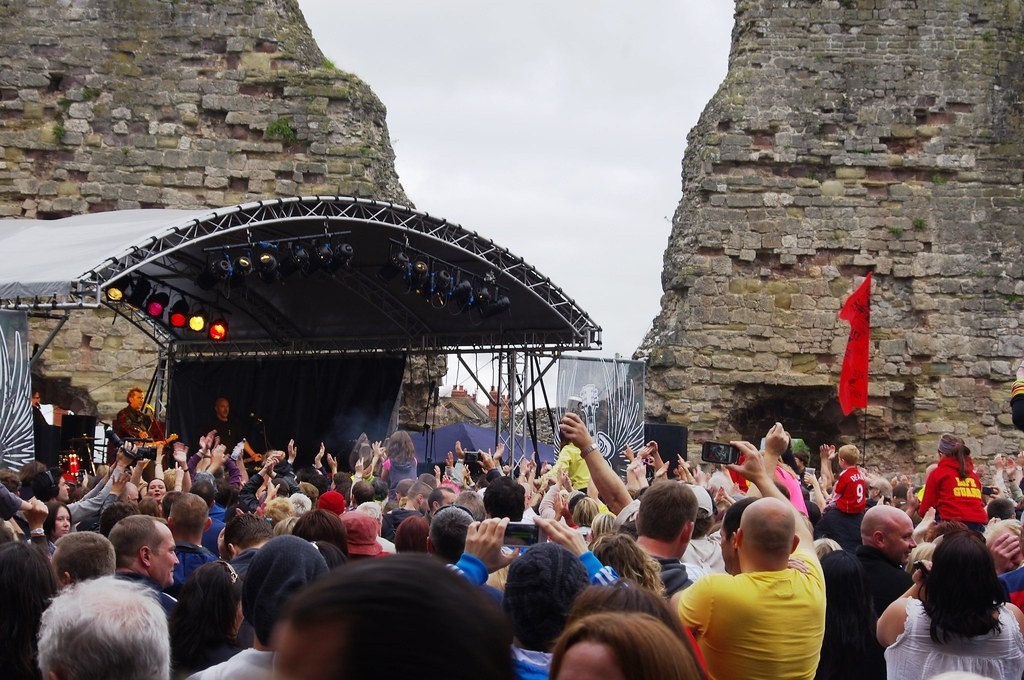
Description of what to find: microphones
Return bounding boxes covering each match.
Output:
[434,387,439,408]
[428,380,436,398]
[250,413,262,422]
[124,437,153,444]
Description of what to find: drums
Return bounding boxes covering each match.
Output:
[59,473,78,487]
[59,449,79,469]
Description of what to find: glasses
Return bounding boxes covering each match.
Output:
[868,485,876,490]
[433,505,474,521]
[215,560,238,585]
[15,530,27,544]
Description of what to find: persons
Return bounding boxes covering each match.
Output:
[32,388,49,462]
[0,413,1024,680]
[117,387,155,444]
[1010,358,1024,433]
[204,397,263,492]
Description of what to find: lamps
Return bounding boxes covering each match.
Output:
[302,246,333,278]
[278,248,309,281]
[169,300,188,327]
[329,243,354,271]
[127,278,152,311]
[145,288,169,319]
[189,310,210,332]
[383,252,511,318]
[106,275,130,301]
[208,319,229,342]
[197,253,277,291]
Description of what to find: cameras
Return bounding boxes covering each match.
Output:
[503,521,547,547]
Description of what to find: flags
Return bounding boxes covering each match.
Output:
[0,308,36,473]
[838,271,872,415]
[554,356,645,485]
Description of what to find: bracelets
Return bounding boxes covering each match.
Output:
[536,491,543,496]
[1008,478,1015,482]
[1018,365,1024,370]
[519,472,525,476]
[184,468,190,472]
[202,454,211,459]
[199,449,206,455]
[270,472,273,474]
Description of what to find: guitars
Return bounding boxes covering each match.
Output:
[244,453,266,464]
[128,433,178,466]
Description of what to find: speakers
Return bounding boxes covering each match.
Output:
[643,423,688,485]
[61,414,96,476]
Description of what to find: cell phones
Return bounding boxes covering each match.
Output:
[465,452,481,462]
[913,560,929,580]
[982,487,994,495]
[702,441,741,465]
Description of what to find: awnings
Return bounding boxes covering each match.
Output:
[409,421,554,466]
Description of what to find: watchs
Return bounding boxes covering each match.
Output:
[30,528,44,535]
[580,443,598,459]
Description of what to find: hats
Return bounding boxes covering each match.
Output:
[242,534,329,633]
[266,477,288,496]
[502,541,590,637]
[317,490,345,515]
[689,485,713,519]
[339,511,384,556]
[794,450,809,466]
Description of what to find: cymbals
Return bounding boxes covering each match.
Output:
[69,436,96,441]
